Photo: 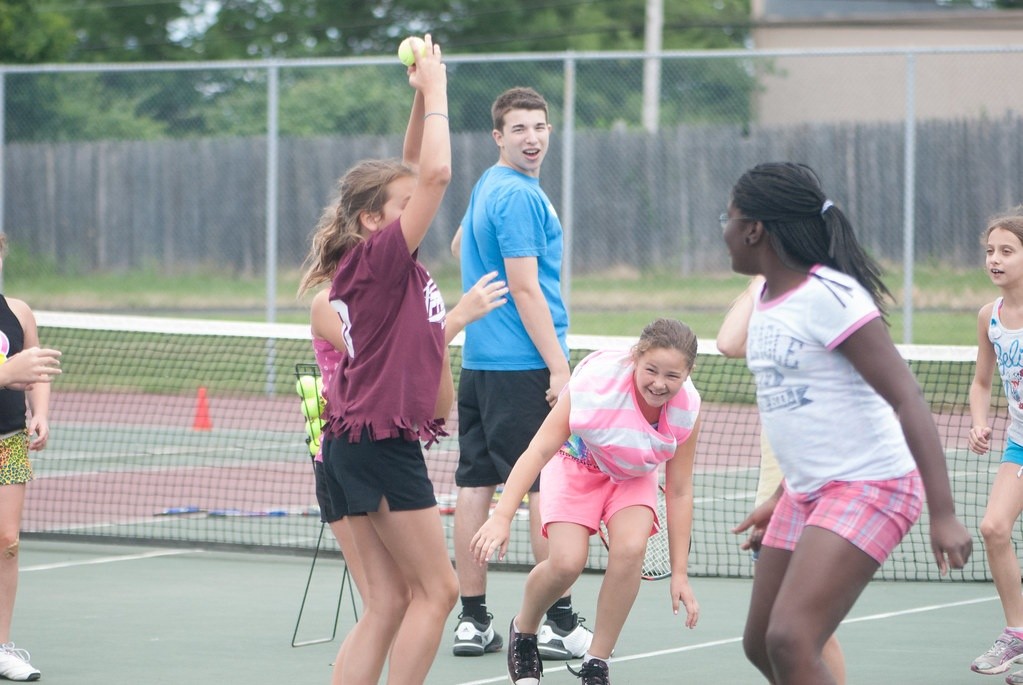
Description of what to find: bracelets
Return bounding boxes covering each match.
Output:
[422,113,450,119]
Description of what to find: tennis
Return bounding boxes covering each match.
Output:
[398,36,427,67]
[305,417,328,439]
[308,439,321,456]
[295,375,325,399]
[300,397,330,418]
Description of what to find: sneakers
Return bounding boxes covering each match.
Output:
[1005,670,1023,685]
[507,615,544,685]
[566,659,611,685]
[970,628,1023,675]
[537,613,614,660]
[0,642,40,681]
[453,611,503,656]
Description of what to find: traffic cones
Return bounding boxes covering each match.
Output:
[190,387,213,430]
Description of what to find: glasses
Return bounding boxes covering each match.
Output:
[719,212,771,236]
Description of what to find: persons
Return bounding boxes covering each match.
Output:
[296,33,509,685]
[0,232,63,682]
[969,216,1023,685]
[451,87,616,659]
[717,160,973,685]
[470,317,702,685]
